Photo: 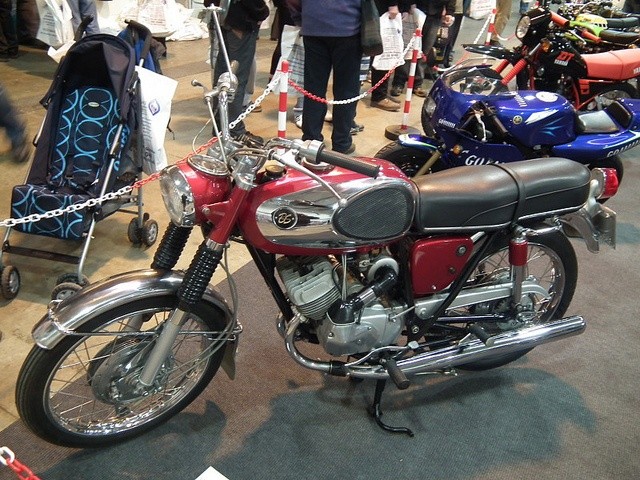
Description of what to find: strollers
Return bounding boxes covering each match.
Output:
[1,15,165,300]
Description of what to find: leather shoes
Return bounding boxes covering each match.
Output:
[370,98,401,111]
[387,95,401,104]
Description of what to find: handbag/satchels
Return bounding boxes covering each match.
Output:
[96,0,210,43]
[401,7,426,60]
[371,12,406,71]
[135,66,179,175]
[272,25,305,98]
[469,1,497,20]
[361,0,382,55]
[35,0,75,47]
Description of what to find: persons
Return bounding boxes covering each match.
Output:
[242,57,262,114]
[371,0,416,111]
[0,0,20,62]
[0,83,34,163]
[268,0,295,89]
[204,0,269,146]
[293,97,333,128]
[426,0,471,80]
[490,0,512,47]
[287,0,363,155]
[60,0,100,42]
[390,0,457,96]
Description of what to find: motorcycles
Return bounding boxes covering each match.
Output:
[15,1,619,447]
[461,7,640,110]
[563,0,640,18]
[531,0,640,52]
[373,59,640,204]
[556,0,640,34]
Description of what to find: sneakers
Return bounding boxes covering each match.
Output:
[489,39,505,48]
[231,131,264,146]
[293,97,303,111]
[242,101,262,113]
[294,114,303,128]
[411,88,427,97]
[349,127,358,135]
[324,110,333,122]
[351,121,365,130]
[390,86,401,95]
[342,144,355,154]
